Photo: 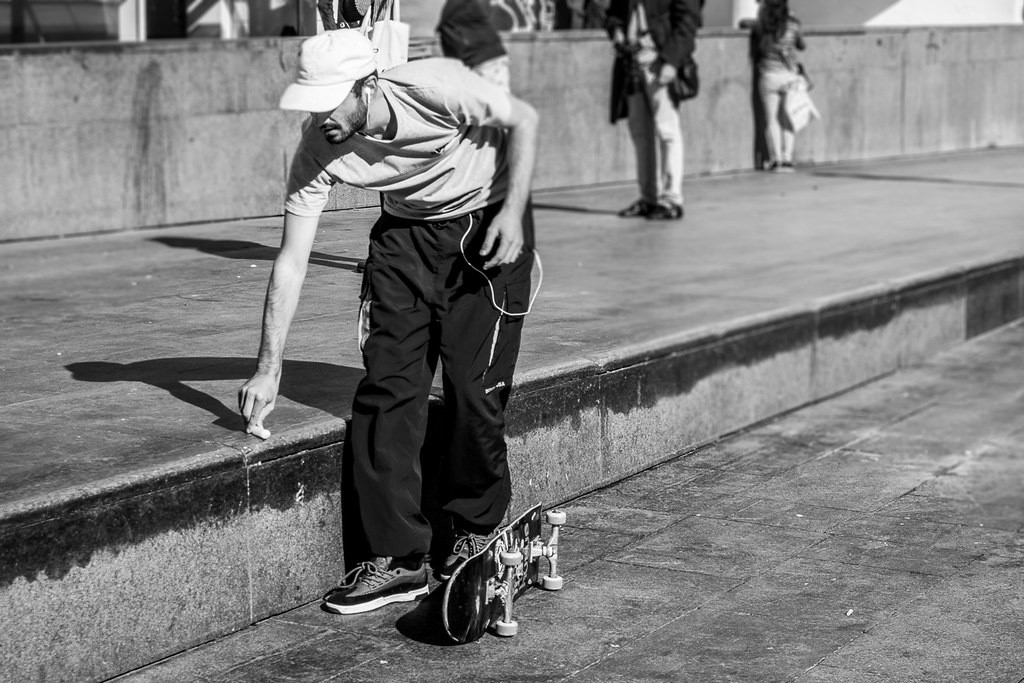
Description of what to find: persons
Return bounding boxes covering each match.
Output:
[599,0,709,220]
[734,1,814,174]
[239,29,544,615]
[436,0,512,99]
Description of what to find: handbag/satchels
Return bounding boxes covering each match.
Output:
[673,61,698,99]
[360,0,414,76]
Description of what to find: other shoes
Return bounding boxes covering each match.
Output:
[767,162,793,176]
[621,203,646,217]
[649,205,671,221]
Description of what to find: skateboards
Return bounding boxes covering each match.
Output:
[441,501,567,642]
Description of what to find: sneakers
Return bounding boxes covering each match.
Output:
[325,549,429,614]
[441,526,498,580]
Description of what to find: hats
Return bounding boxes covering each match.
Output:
[280,29,376,113]
